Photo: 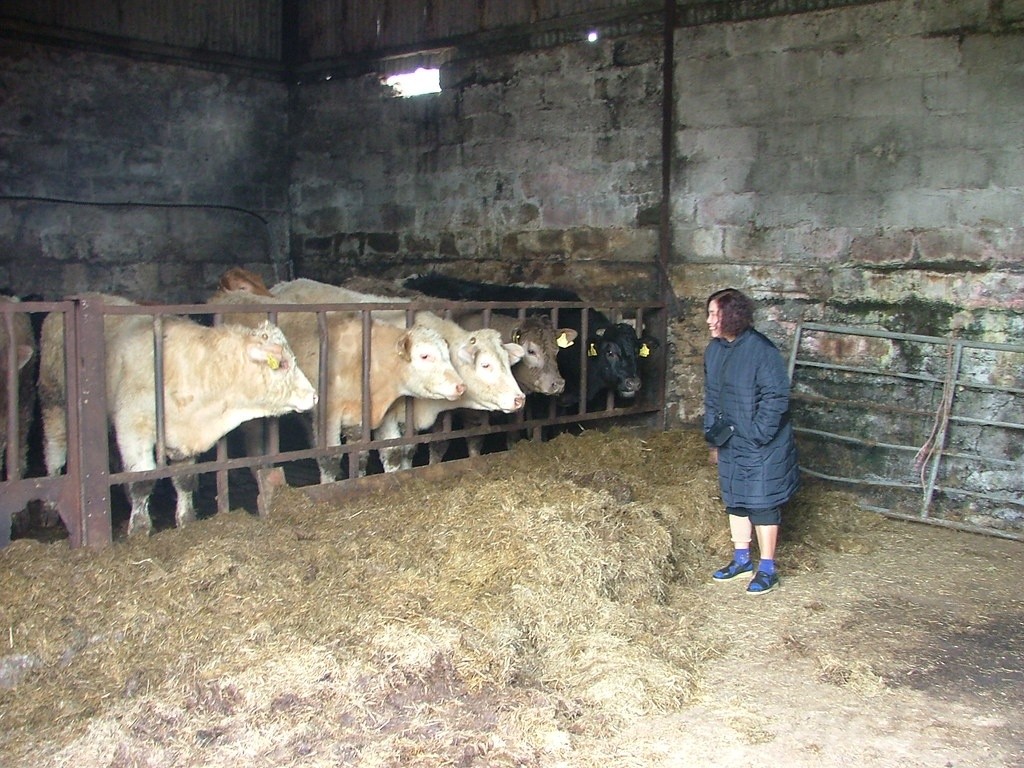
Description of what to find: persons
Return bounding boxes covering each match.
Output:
[702,288,801,596]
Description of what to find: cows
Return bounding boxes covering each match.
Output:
[0,293,41,468]
[405,272,644,415]
[39,289,321,540]
[346,272,577,401]
[206,286,468,485]
[271,274,529,414]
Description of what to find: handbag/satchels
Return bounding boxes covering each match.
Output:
[705,419,735,446]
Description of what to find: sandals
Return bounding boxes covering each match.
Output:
[712,560,753,582]
[747,570,779,595]
